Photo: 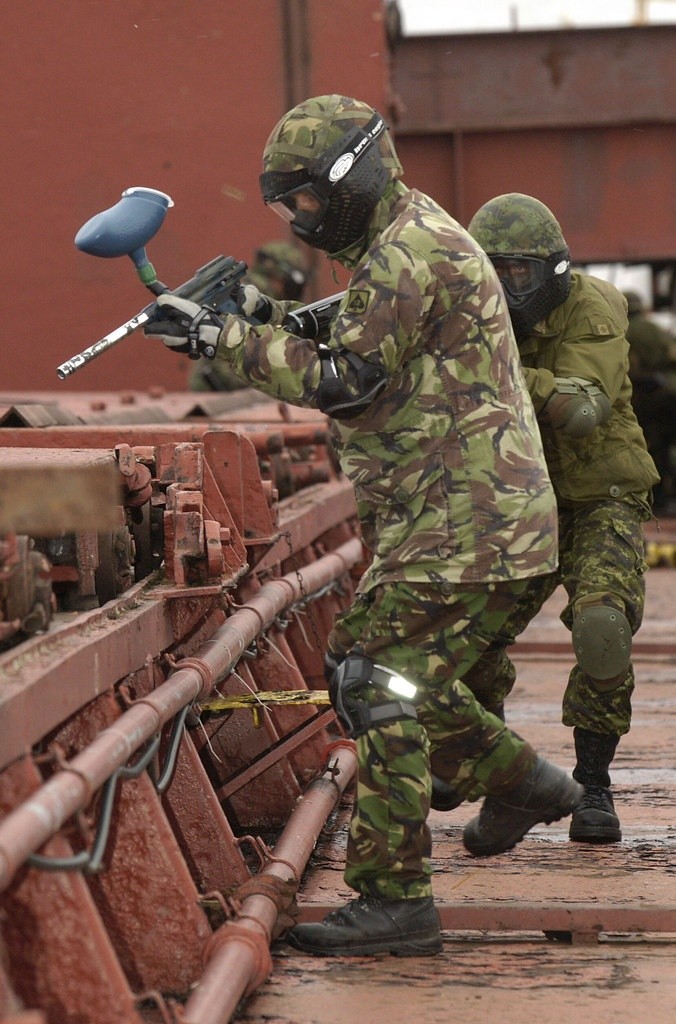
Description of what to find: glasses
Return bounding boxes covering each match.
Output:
[490,254,547,296]
[266,182,329,232]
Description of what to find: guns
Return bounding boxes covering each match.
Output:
[55,253,264,381]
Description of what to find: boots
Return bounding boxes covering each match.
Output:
[568,726,622,841]
[429,701,506,812]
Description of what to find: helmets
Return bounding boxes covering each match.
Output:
[622,292,643,314]
[466,192,572,264]
[259,94,404,201]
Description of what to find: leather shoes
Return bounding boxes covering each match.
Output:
[288,894,445,958]
[463,756,585,857]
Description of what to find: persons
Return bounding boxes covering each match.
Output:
[190,241,311,391]
[430,191,658,842]
[621,292,676,514]
[144,93,583,958]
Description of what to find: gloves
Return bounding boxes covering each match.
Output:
[142,294,224,360]
[236,284,272,324]
